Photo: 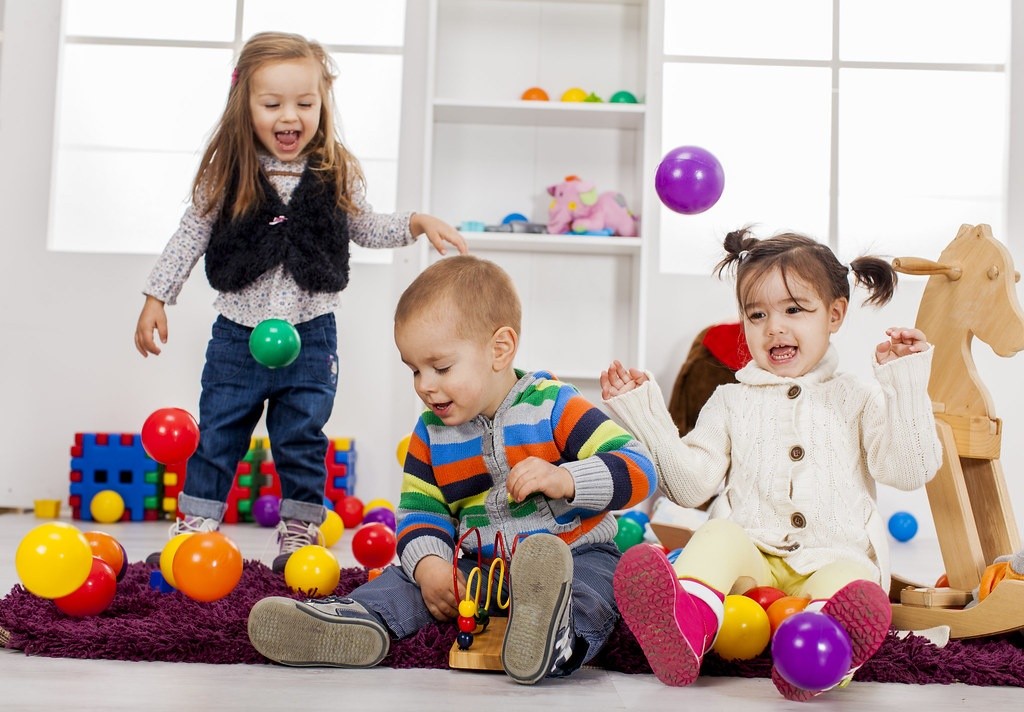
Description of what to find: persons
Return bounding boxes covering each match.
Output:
[598,222,946,701]
[248,254,660,687]
[132,28,470,581]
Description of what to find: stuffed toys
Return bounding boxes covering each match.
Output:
[545,176,636,236]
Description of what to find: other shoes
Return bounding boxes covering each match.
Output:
[248,594,389,669]
[613,542,706,687]
[177,516,220,535]
[771,579,893,702]
[500,533,575,684]
[272,519,319,570]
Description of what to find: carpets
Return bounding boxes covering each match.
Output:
[0,552,1024,690]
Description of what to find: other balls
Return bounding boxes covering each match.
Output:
[285,495,397,596]
[656,146,726,214]
[253,495,280,528]
[501,212,527,225]
[248,319,301,368]
[611,510,686,565]
[520,87,640,107]
[90,489,125,523]
[140,407,201,466]
[711,584,852,691]
[888,513,917,541]
[14,518,243,618]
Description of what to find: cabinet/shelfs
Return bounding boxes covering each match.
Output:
[416,1,666,422]
